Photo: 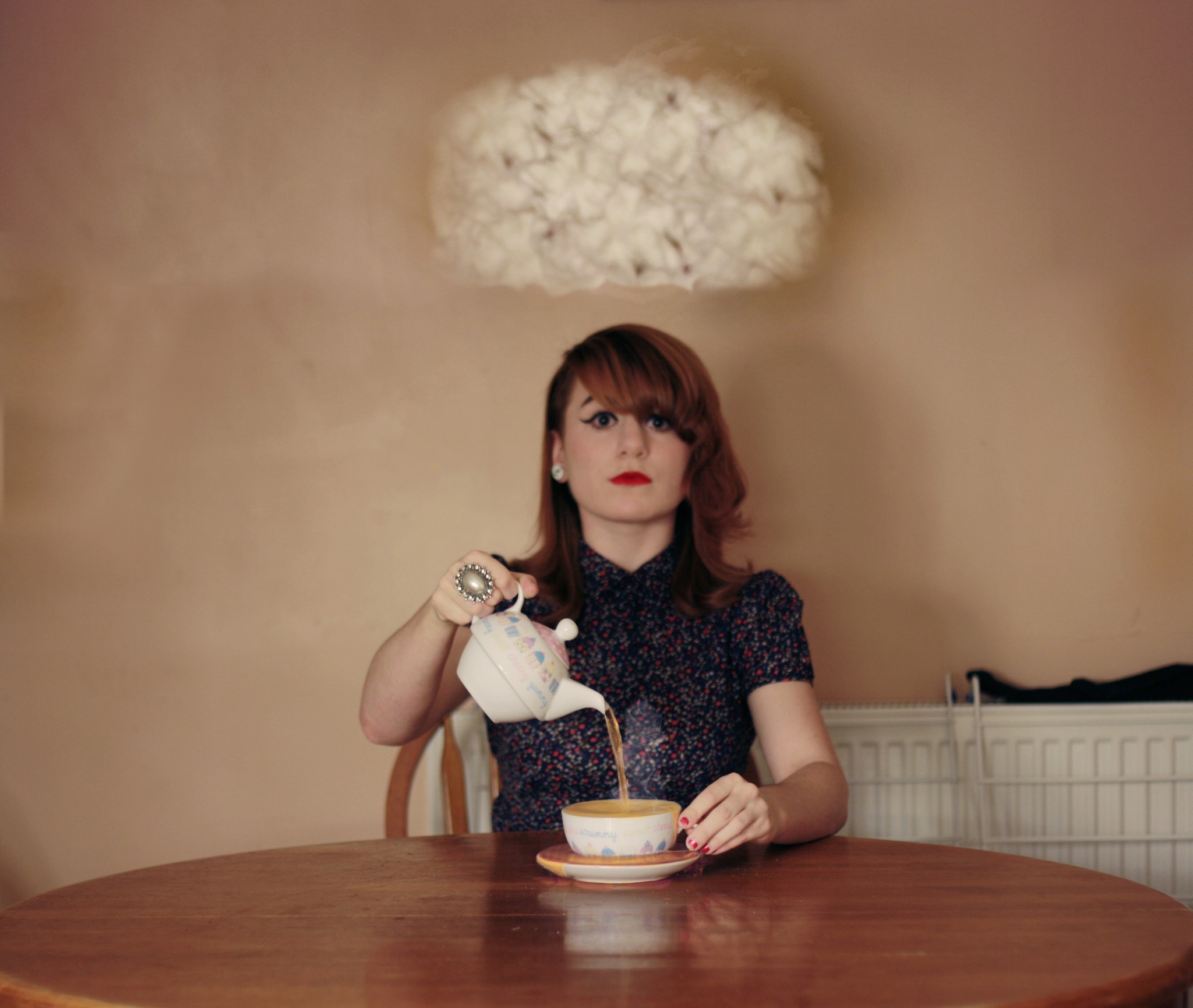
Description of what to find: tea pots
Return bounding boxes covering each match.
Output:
[456,581,606,723]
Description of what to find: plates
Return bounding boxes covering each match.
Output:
[537,844,700,884]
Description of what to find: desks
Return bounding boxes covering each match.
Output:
[0,830,1193,1008]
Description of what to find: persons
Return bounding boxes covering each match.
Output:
[359,322,848,857]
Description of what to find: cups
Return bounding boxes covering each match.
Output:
[561,799,698,856]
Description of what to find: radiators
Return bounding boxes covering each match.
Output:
[424,704,1193,912]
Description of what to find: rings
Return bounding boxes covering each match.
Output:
[453,563,495,605]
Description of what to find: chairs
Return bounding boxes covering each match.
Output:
[385,694,503,842]
